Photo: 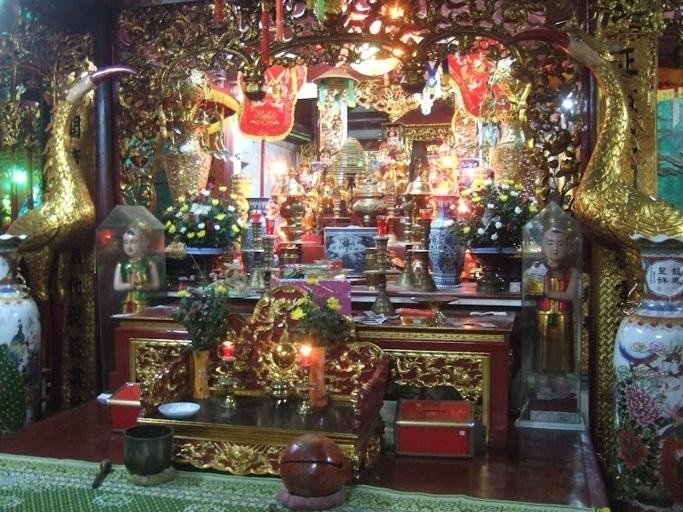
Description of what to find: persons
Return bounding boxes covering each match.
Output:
[112,223,161,317]
[267,156,336,235]
[521,226,578,400]
[371,128,463,202]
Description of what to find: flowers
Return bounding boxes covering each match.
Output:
[164,184,249,248]
[289,276,351,347]
[171,283,231,357]
[449,178,539,246]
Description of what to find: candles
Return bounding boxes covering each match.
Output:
[222,345,234,357]
[420,213,430,219]
[265,217,275,235]
[252,213,261,223]
[376,215,389,235]
[301,354,311,367]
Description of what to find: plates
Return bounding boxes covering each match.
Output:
[158,398,201,419]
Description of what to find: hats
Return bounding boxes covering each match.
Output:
[312,67,360,89]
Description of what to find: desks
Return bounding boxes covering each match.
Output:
[1,299,613,512]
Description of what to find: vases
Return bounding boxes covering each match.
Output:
[191,349,210,399]
[239,198,276,281]
[183,249,226,283]
[428,202,464,289]
[0,233,43,437]
[468,247,516,292]
[308,347,328,408]
[612,233,683,512]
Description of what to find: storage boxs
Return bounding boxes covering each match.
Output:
[393,397,477,459]
[105,381,142,434]
